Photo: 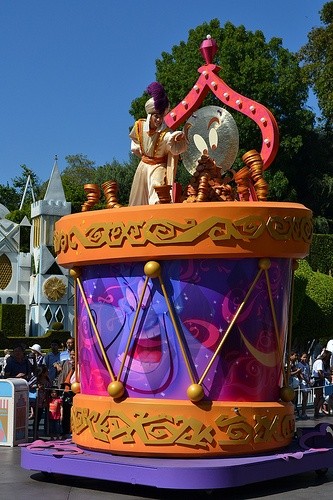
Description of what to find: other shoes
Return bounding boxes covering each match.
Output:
[28,414,34,420]
[56,437,59,440]
[319,411,323,413]
[50,437,55,441]
[323,410,329,415]
[296,416,299,419]
[301,414,307,420]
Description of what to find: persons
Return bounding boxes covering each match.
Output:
[0,337,75,441]
[129,97,187,206]
[284,347,333,419]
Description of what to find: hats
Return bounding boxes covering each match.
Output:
[29,344,43,356]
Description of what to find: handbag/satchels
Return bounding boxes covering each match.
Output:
[292,375,300,388]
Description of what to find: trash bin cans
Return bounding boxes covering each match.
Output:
[0,378,29,448]
